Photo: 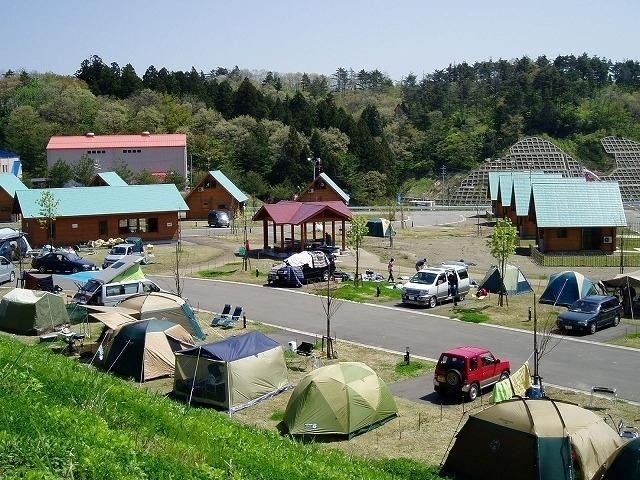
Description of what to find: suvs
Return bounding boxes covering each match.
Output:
[433,346,511,402]
[556,294,625,334]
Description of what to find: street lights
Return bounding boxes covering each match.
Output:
[307,156,321,180]
[207,155,212,171]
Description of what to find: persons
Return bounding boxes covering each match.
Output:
[325,231,332,245]
[415,258,427,272]
[624,286,636,315]
[388,257,396,283]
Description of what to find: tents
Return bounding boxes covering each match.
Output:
[440,392,626,479]
[364,217,396,237]
[478,261,533,294]
[79,290,206,341]
[283,360,399,440]
[1,286,71,334]
[539,269,600,308]
[588,434,638,480]
[88,312,195,382]
[171,329,292,415]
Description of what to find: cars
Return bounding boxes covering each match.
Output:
[0,232,144,283]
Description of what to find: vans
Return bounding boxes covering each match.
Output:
[208,209,230,228]
[71,279,170,312]
[401,260,478,309]
[266,245,339,288]
